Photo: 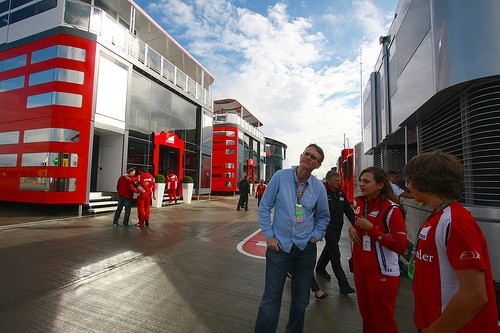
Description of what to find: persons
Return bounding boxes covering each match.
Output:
[405,151,500,333]
[165,169,178,205]
[122,164,157,226]
[236,174,251,211]
[386,169,414,202]
[253,144,330,333]
[318,171,356,294]
[255,179,268,206]
[345,168,406,333]
[112,169,145,227]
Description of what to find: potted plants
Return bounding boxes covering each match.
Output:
[182,176,194,203]
[150,174,166,208]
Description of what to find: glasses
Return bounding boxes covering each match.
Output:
[303,152,321,163]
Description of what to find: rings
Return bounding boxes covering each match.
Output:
[353,235,354,237]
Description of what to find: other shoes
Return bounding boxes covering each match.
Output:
[315,267,331,279]
[135,223,144,227]
[339,286,355,294]
[241,206,245,208]
[314,292,327,299]
[113,223,119,226]
[145,222,149,226]
[237,208,242,211]
[245,209,249,211]
[122,224,132,227]
